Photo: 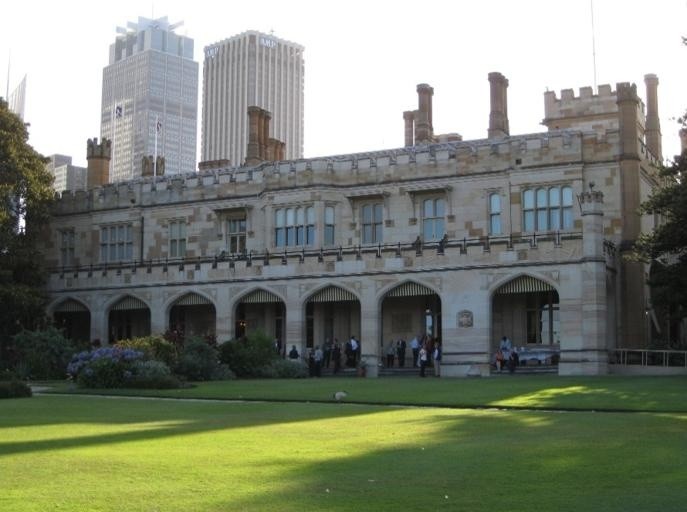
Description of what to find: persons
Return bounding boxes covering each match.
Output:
[386,339,395,368]
[499,336,512,352]
[272,336,279,353]
[288,345,298,360]
[507,348,518,374]
[306,334,360,377]
[496,348,504,372]
[396,337,405,368]
[410,332,441,378]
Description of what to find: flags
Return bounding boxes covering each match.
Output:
[156,121,162,134]
[115,105,123,119]
